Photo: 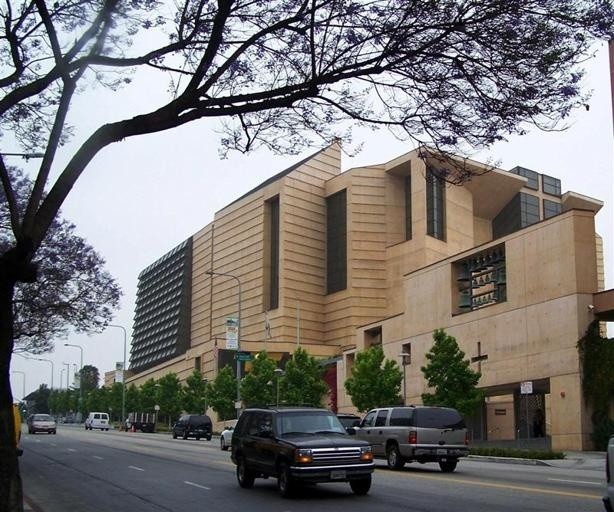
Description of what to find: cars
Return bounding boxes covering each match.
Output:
[219,419,238,450]
[601,433,613,512]
[26,413,56,435]
[62,416,72,423]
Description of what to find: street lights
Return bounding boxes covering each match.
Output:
[273,367,283,407]
[397,350,411,406]
[204,271,241,420]
[94,323,125,423]
[9,343,83,401]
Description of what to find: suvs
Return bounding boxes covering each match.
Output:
[229,405,375,500]
[335,412,362,430]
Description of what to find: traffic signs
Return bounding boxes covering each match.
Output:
[232,352,251,361]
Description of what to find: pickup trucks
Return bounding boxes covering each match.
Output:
[124,412,154,432]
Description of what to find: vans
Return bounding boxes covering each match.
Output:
[355,404,472,474]
[83,412,110,431]
[170,413,213,440]
